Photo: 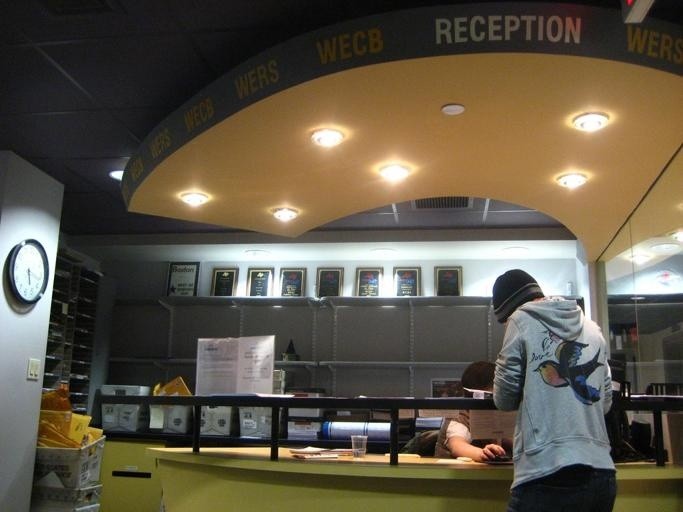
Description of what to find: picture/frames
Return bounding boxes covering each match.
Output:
[209,267,239,297]
[279,267,307,296]
[355,267,384,297]
[433,265,465,295]
[392,267,421,297]
[315,267,344,298]
[165,260,201,297]
[246,267,274,297]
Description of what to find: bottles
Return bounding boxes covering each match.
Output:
[59,380,72,412]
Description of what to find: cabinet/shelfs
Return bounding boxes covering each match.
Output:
[319,295,497,370]
[107,296,317,369]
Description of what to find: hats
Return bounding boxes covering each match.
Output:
[493,269,545,322]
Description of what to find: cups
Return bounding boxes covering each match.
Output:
[351,435,368,458]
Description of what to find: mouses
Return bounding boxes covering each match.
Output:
[497,452,511,461]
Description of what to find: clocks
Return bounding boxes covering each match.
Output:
[6,237,49,306]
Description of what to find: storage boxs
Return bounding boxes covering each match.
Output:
[97,384,152,432]
[30,483,103,512]
[285,386,327,417]
[34,431,106,484]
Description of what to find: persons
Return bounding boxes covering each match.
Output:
[493,269,617,512]
[434,362,514,462]
